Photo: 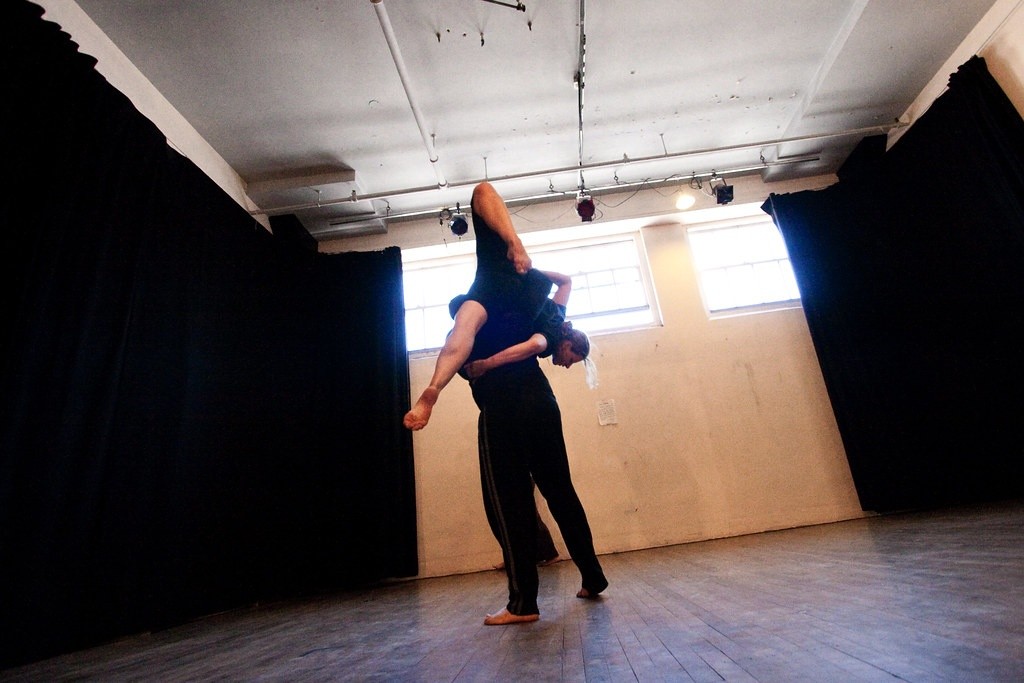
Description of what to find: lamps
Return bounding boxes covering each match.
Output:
[575,193,594,221]
[709,177,734,205]
[449,214,468,237]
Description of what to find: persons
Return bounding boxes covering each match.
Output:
[402,182,610,626]
